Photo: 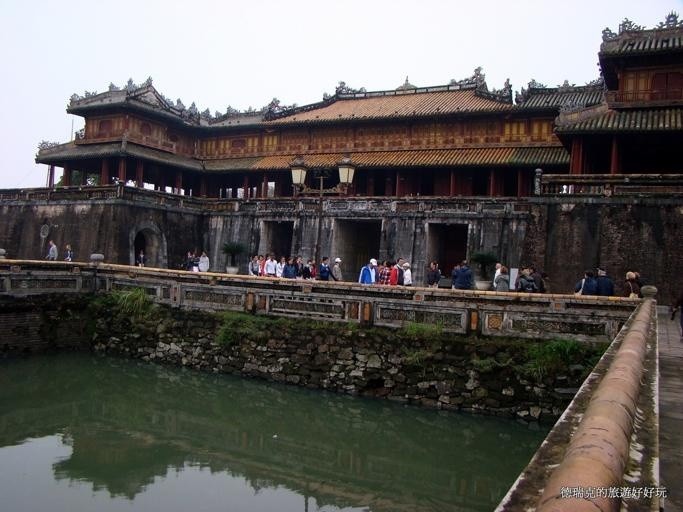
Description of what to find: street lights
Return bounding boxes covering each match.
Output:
[290,152,357,282]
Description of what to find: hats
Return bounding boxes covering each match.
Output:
[369,257,378,267]
[402,261,410,267]
[334,257,342,263]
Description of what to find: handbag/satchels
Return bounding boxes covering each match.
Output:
[250,260,259,277]
[573,278,586,296]
[625,281,639,300]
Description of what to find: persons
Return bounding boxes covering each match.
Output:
[574,269,643,297]
[45,239,58,261]
[182,251,210,272]
[136,248,147,267]
[673,291,683,337]
[247,253,549,293]
[63,244,73,262]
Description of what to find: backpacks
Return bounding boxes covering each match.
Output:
[524,279,534,293]
